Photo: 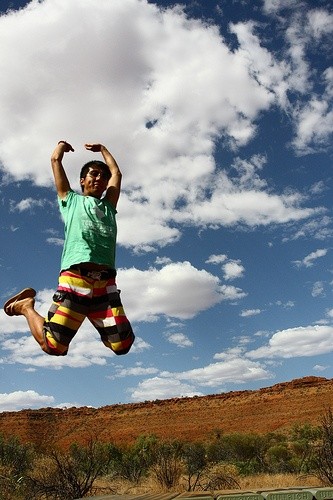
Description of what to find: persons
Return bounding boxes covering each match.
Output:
[3,140,135,356]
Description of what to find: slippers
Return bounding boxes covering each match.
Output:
[3,287,36,316]
